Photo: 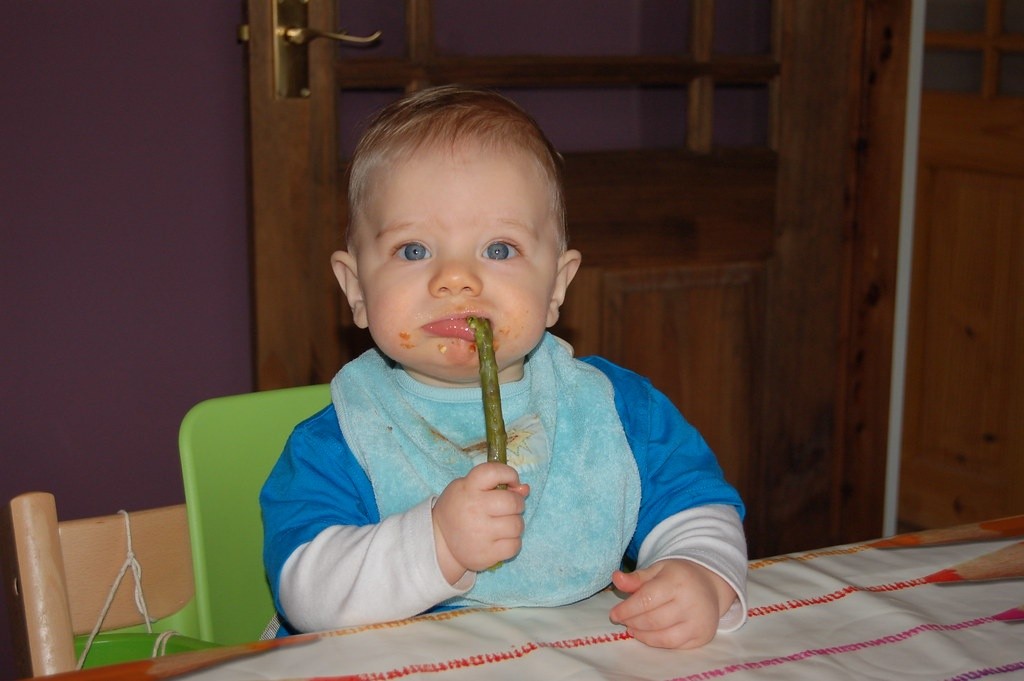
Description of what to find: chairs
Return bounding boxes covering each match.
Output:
[10,491,192,677]
[178,385,370,681]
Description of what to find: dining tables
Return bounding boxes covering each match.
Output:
[56,513,1024,681]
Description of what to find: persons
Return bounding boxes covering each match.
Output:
[254,83,748,651]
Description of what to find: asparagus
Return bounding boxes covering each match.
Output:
[467,316,507,570]
